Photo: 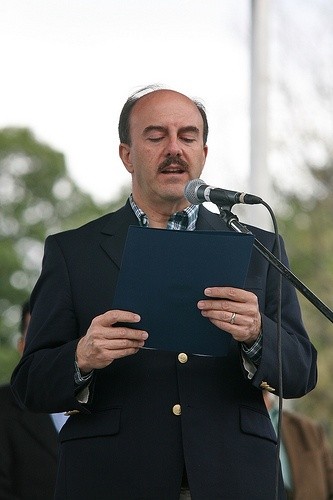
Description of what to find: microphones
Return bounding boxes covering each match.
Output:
[185,179,261,205]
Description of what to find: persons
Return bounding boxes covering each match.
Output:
[0,300,70,500]
[10,85,318,500]
[262,390,333,500]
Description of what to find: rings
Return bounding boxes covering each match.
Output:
[230,313,236,324]
